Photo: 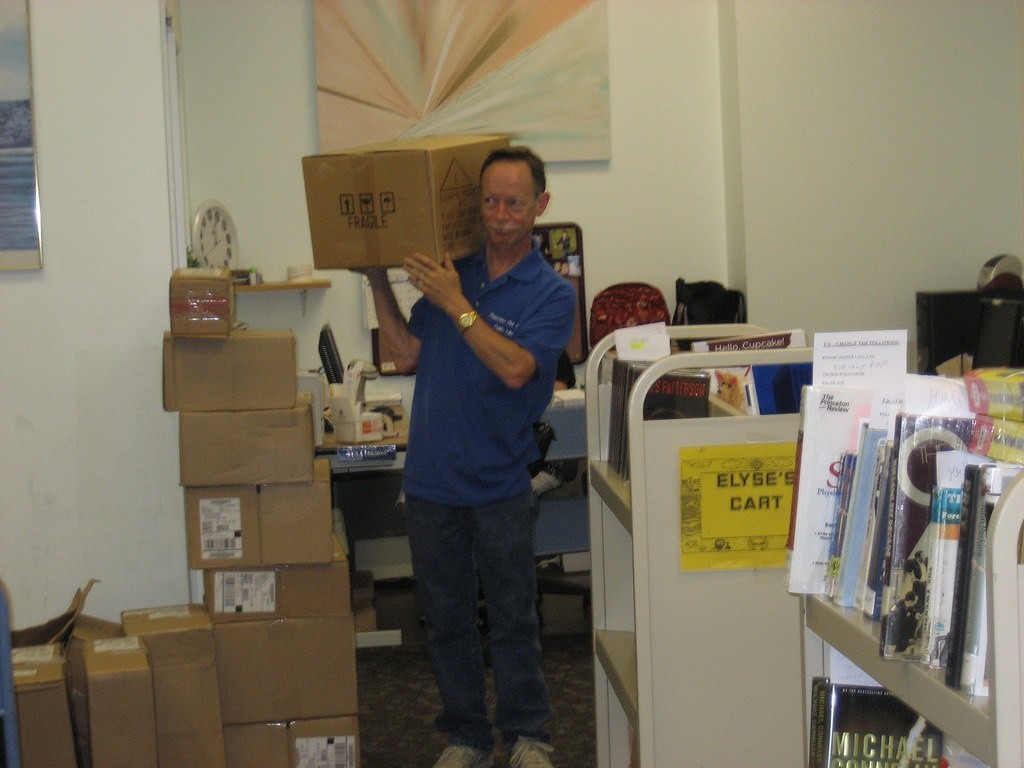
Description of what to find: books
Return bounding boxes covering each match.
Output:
[691,329,813,415]
[809,677,944,768]
[607,358,711,482]
[784,385,1024,697]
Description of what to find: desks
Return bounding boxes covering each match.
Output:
[314,405,410,536]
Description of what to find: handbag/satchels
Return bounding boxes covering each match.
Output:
[671,277,747,352]
[589,282,671,351]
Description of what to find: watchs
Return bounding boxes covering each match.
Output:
[456,310,480,335]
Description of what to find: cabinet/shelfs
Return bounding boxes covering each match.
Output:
[800,466,1024,768]
[584,323,813,768]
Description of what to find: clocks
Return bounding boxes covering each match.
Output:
[192,198,240,271]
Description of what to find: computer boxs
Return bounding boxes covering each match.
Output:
[916,287,1024,375]
[295,373,324,447]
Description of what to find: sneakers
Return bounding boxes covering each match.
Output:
[508,736,555,768]
[431,743,487,768]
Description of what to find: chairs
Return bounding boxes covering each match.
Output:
[416,387,587,632]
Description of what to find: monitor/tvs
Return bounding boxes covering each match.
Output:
[971,297,1024,369]
[317,324,345,385]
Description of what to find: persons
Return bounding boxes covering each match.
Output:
[355,146,577,768]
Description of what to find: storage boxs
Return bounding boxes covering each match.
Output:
[301,134,512,272]
[351,569,378,632]
[12,269,360,768]
